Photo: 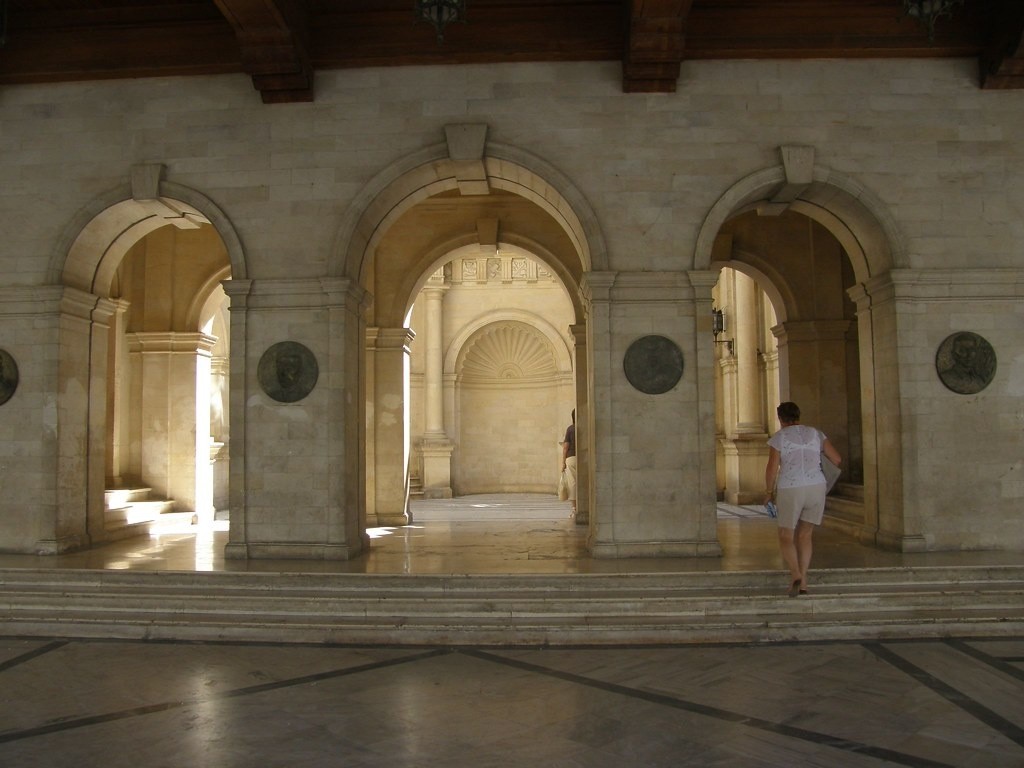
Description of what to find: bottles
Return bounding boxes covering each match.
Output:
[767,501,777,519]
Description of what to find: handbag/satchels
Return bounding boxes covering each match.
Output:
[817,431,842,495]
[557,471,568,502]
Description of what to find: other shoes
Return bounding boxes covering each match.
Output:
[568,510,576,519]
[788,574,801,597]
[800,584,808,594]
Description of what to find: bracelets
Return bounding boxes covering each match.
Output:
[766,489,774,494]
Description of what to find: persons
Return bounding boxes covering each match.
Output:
[560,409,575,518]
[765,401,842,599]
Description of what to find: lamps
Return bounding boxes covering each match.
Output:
[712,307,734,355]
[895,0,965,47]
[410,0,473,48]
